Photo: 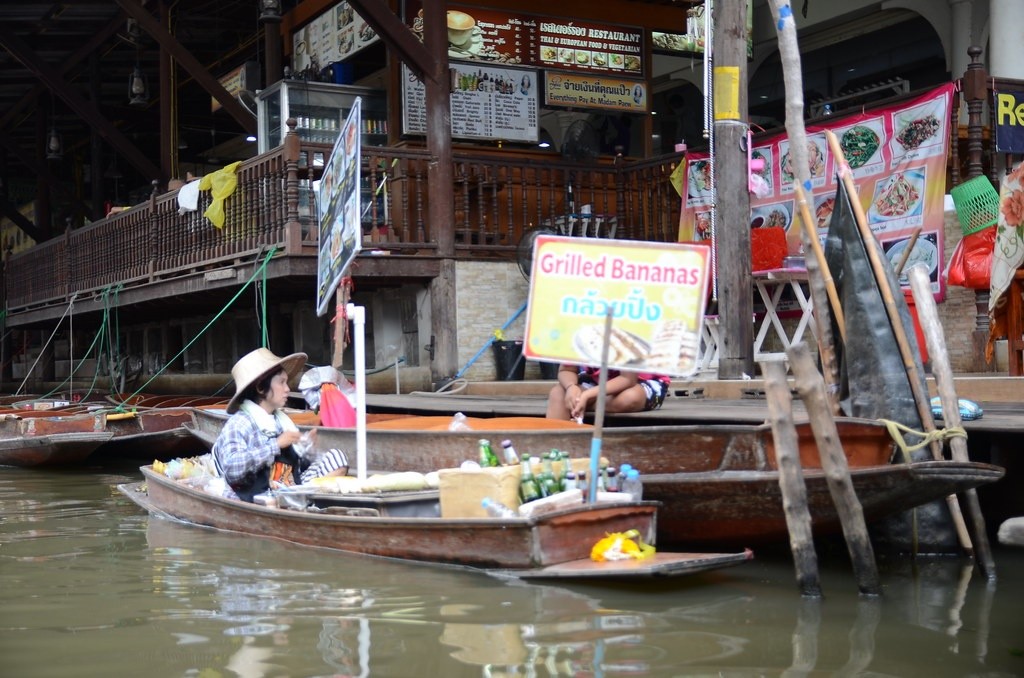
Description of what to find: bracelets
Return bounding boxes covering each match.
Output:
[565,383,578,391]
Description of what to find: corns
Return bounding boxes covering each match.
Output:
[309,470,440,492]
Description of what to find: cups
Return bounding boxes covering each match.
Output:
[447,10,482,46]
[675,143,687,152]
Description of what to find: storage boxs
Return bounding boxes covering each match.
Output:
[785,255,806,270]
[438,456,609,518]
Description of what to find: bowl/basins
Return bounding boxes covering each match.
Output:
[751,215,765,229]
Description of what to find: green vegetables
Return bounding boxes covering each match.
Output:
[841,126,879,168]
[752,150,789,169]
[696,160,707,171]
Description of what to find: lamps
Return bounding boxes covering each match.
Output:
[823,105,832,117]
[257,0,283,24]
[44,93,63,154]
[128,36,150,106]
[239,89,258,119]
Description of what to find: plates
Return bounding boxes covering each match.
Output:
[448,33,483,57]
[686,106,940,286]
[337,40,353,57]
[541,45,643,74]
[355,20,379,47]
[296,41,307,56]
[318,116,357,303]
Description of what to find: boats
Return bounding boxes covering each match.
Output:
[0,397,231,469]
[181,402,1005,543]
[119,450,755,580]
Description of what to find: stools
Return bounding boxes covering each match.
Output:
[700,313,757,370]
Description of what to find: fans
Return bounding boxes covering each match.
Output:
[514,225,563,284]
[560,118,599,171]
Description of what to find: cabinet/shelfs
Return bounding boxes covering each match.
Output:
[253,77,387,241]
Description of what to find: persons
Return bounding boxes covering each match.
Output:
[546,363,670,423]
[634,86,642,104]
[520,74,532,96]
[211,347,349,504]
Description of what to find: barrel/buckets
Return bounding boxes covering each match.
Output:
[493,339,526,381]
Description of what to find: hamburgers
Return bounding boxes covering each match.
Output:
[447,10,475,52]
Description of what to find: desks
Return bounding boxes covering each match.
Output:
[751,265,818,365]
[309,489,440,517]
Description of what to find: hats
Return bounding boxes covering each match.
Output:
[226,347,308,415]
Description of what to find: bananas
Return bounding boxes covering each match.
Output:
[153,457,202,480]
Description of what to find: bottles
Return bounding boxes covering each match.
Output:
[478,439,500,468]
[458,69,516,94]
[560,451,572,491]
[599,456,644,502]
[822,104,832,116]
[578,471,588,502]
[501,440,520,466]
[481,498,515,518]
[565,473,574,491]
[566,183,575,214]
[519,453,541,503]
[539,452,560,498]
